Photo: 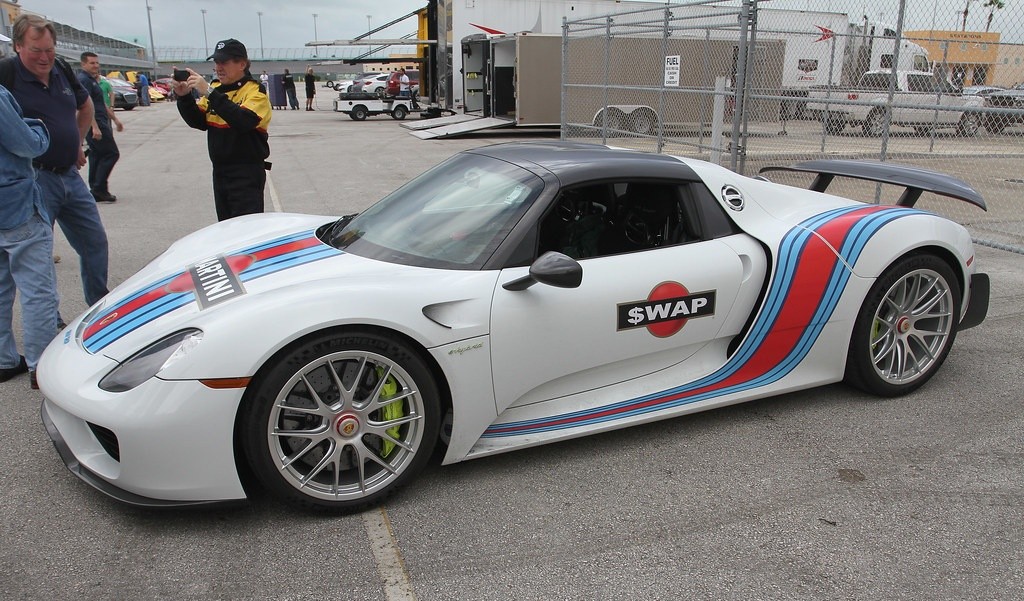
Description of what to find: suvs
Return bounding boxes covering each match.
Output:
[981,83,1024,135]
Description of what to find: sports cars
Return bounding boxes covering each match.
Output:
[35,139,989,520]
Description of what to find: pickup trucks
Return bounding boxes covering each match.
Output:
[806,68,985,139]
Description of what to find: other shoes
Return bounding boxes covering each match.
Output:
[95,192,116,201]
[58,320,67,331]
[306,108,308,111]
[0,355,27,382]
[52,256,61,262]
[31,371,39,389]
[309,108,315,111]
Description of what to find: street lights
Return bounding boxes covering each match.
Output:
[88,5,95,32]
[366,15,372,57]
[200,9,208,57]
[256,12,264,61]
[311,14,319,55]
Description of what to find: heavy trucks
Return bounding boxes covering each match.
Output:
[305,1,936,114]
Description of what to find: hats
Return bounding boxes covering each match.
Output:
[206,38,247,61]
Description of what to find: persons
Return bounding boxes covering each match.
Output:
[171,38,271,221]
[76,52,119,202]
[305,64,315,111]
[398,68,409,95]
[0,14,110,333]
[94,69,114,116]
[0,84,59,390]
[260,71,268,88]
[134,71,153,106]
[282,69,299,110]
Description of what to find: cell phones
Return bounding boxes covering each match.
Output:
[173,69,190,81]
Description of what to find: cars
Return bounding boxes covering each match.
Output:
[103,72,173,111]
[963,85,1006,98]
[338,69,419,102]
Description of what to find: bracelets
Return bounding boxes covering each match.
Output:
[206,89,214,97]
[110,106,114,107]
